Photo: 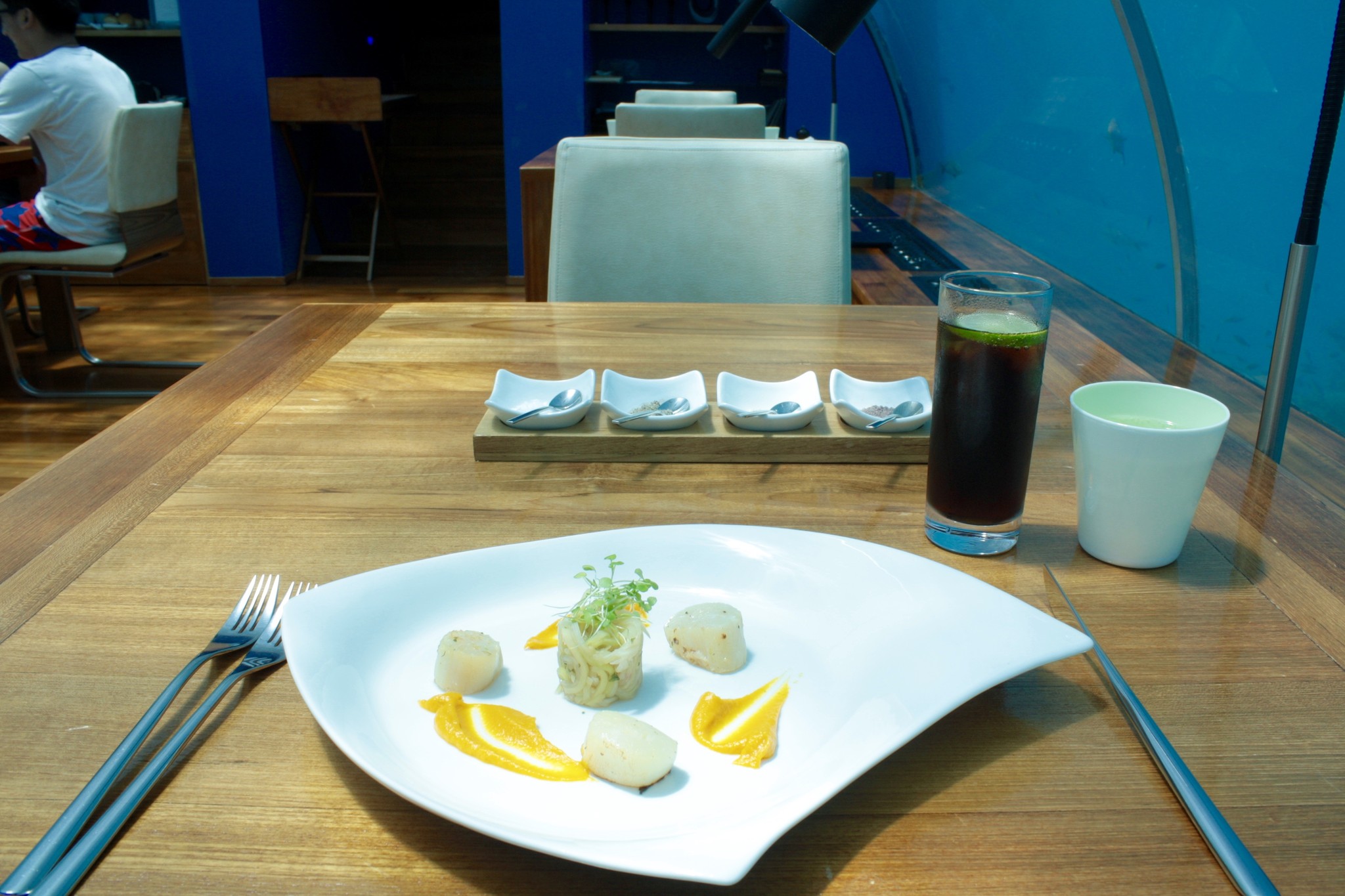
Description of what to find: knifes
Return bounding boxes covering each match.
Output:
[1041,563,1280,896]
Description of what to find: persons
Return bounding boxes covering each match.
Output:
[0,0,138,311]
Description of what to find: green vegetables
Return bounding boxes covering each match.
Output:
[551,554,660,649]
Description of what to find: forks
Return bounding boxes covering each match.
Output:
[27,582,319,895]
[1,574,282,894]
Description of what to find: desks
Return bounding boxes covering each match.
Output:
[520,143,555,303]
[0,144,78,353]
[382,95,411,269]
[0,303,1345,896]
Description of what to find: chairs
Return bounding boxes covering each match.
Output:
[0,100,204,399]
[546,88,851,303]
[265,75,404,282]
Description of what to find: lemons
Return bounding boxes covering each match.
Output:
[948,313,1050,347]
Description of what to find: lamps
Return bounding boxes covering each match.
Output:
[770,0,878,141]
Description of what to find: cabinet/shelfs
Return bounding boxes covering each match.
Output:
[70,29,205,288]
[587,21,782,86]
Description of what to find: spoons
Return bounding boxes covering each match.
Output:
[507,389,582,424]
[736,401,802,419]
[612,397,689,424]
[865,401,923,429]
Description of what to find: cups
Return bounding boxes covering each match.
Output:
[765,126,780,139]
[922,268,1053,556]
[606,120,615,136]
[1069,379,1231,568]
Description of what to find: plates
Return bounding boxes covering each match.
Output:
[485,368,596,431]
[716,371,824,431]
[830,369,933,432]
[599,369,709,430]
[278,519,1093,886]
